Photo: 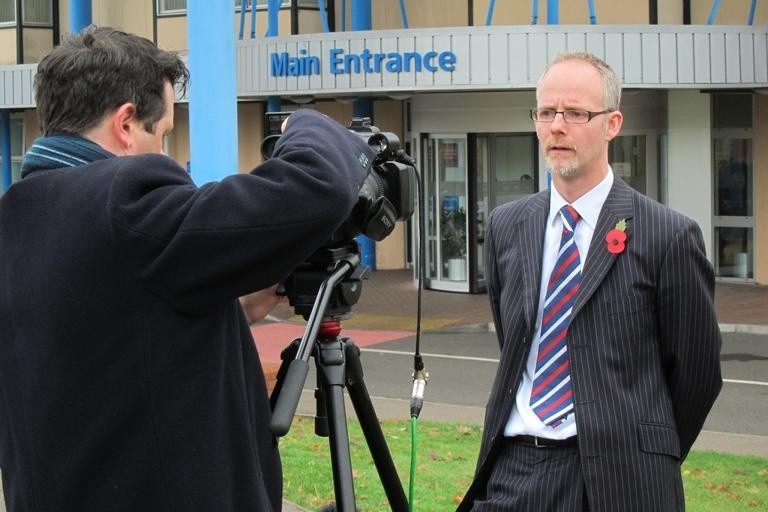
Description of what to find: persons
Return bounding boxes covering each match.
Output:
[1,26,374,512]
[457,53,722,512]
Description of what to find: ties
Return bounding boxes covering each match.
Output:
[528,204,583,429]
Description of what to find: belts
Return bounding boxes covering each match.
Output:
[506,429,577,448]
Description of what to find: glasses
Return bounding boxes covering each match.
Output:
[527,105,613,125]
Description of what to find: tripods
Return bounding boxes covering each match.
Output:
[269,336,409,512]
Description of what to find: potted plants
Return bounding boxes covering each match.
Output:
[440,206,466,282]
